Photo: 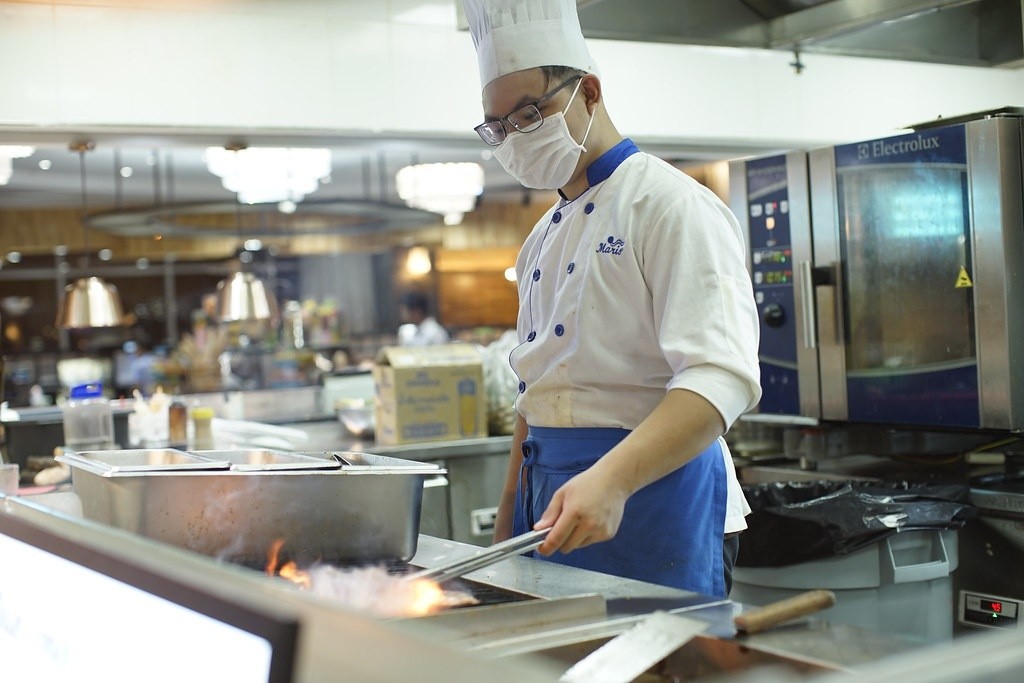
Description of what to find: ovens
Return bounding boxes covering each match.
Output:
[728,120,1024,431]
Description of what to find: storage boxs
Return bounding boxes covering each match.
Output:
[376,344,487,447]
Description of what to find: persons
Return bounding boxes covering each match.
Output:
[397,292,449,346]
[461,1,764,599]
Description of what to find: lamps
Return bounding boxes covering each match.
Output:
[214,270,270,322]
[55,273,123,329]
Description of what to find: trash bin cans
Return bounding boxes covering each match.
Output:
[728,480,977,646]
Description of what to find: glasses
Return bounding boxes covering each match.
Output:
[472,76,581,147]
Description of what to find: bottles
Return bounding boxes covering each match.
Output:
[62,382,115,484]
[191,408,213,450]
[169,387,187,451]
[150,386,170,414]
[459,379,477,436]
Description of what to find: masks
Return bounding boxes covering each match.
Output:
[492,78,596,190]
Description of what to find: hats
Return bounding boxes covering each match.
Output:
[462,0,594,94]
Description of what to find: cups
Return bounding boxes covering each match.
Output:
[0,464,19,494]
